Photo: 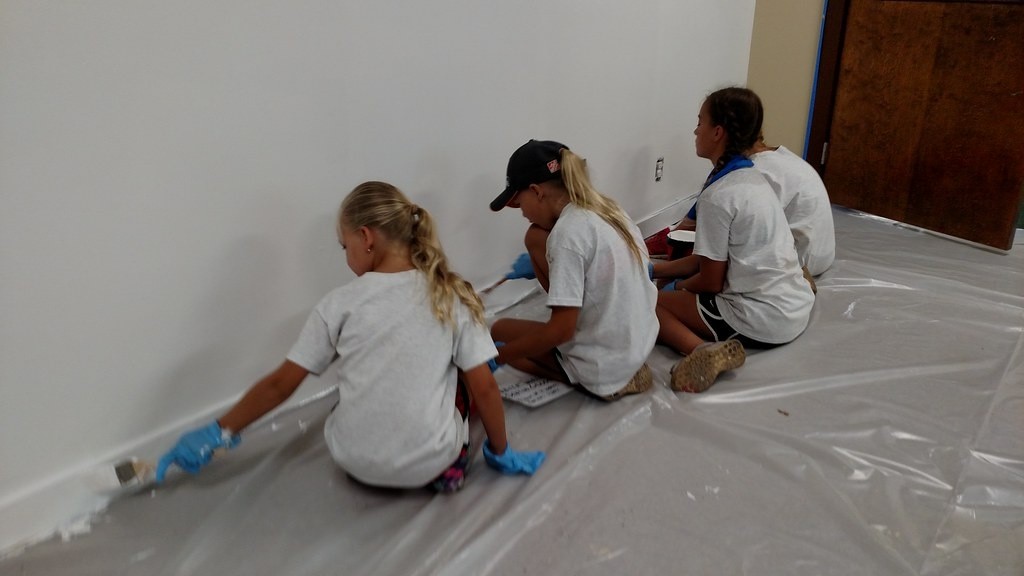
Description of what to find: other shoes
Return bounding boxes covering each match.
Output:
[802,266,817,293]
[671,339,746,393]
[600,362,653,403]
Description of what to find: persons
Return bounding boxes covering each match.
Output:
[154,182,547,497]
[666,129,837,275]
[489,139,659,402]
[647,87,818,394]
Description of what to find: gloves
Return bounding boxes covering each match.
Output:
[504,253,536,280]
[486,341,506,373]
[648,260,654,282]
[482,438,544,476]
[660,279,681,291]
[156,419,241,485]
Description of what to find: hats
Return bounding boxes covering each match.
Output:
[490,139,569,212]
